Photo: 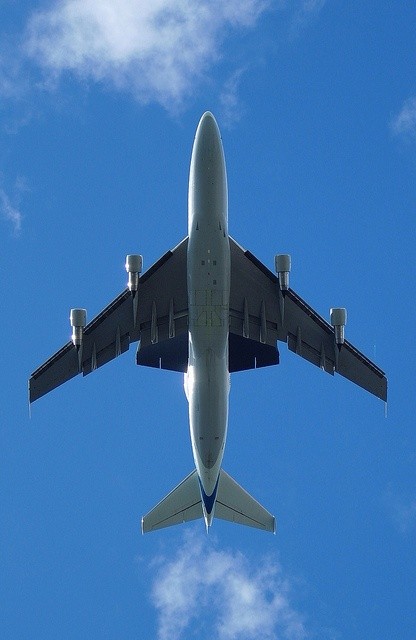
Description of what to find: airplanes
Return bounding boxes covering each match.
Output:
[28,110,388,536]
